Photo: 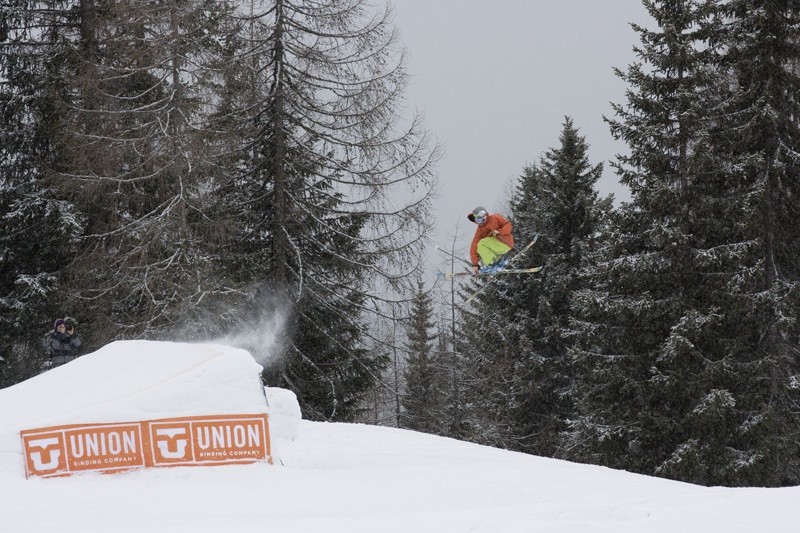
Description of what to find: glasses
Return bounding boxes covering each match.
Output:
[474,217,486,223]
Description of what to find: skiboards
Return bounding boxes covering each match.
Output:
[436,234,543,307]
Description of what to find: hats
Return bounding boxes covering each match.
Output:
[472,207,487,218]
[54,318,64,326]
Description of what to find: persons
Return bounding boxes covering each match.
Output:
[467,205,515,276]
[44,316,82,370]
